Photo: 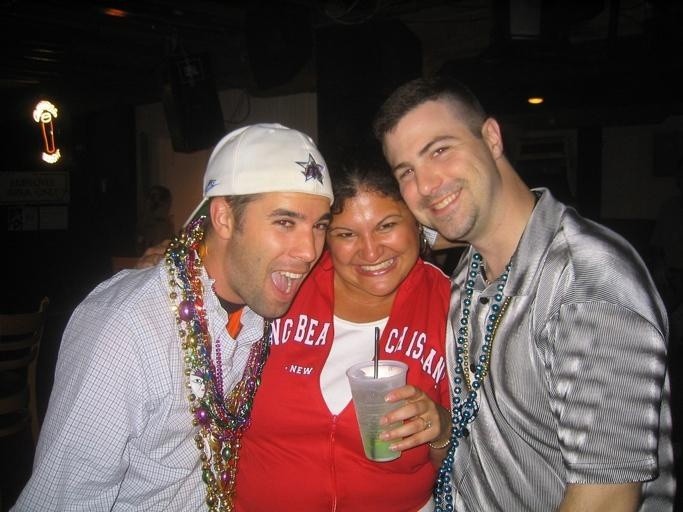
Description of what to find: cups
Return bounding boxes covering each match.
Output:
[346,359,410,465]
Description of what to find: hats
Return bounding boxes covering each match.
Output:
[182,124,334,228]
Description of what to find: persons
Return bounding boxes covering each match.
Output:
[371,77,675,511]
[229,156,463,511]
[5,121,335,511]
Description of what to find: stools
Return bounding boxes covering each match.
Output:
[505,129,579,200]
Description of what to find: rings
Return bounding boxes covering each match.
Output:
[417,414,431,430]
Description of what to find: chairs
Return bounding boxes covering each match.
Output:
[0,295,50,456]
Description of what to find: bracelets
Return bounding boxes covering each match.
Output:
[424,407,453,449]
